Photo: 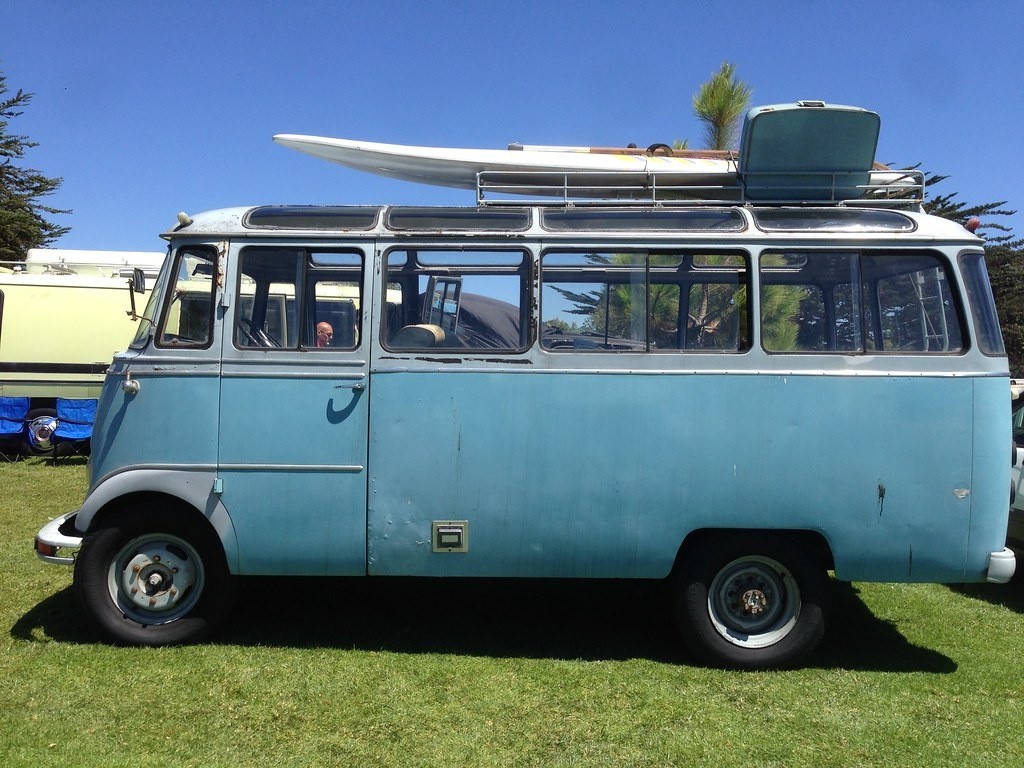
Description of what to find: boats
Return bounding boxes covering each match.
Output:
[269,132,915,197]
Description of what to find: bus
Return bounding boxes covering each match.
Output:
[29,169,1016,670]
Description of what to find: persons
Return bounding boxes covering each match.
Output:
[317,322,333,347]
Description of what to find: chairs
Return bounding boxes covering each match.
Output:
[389,323,446,349]
[0,395,31,463]
[49,397,100,468]
